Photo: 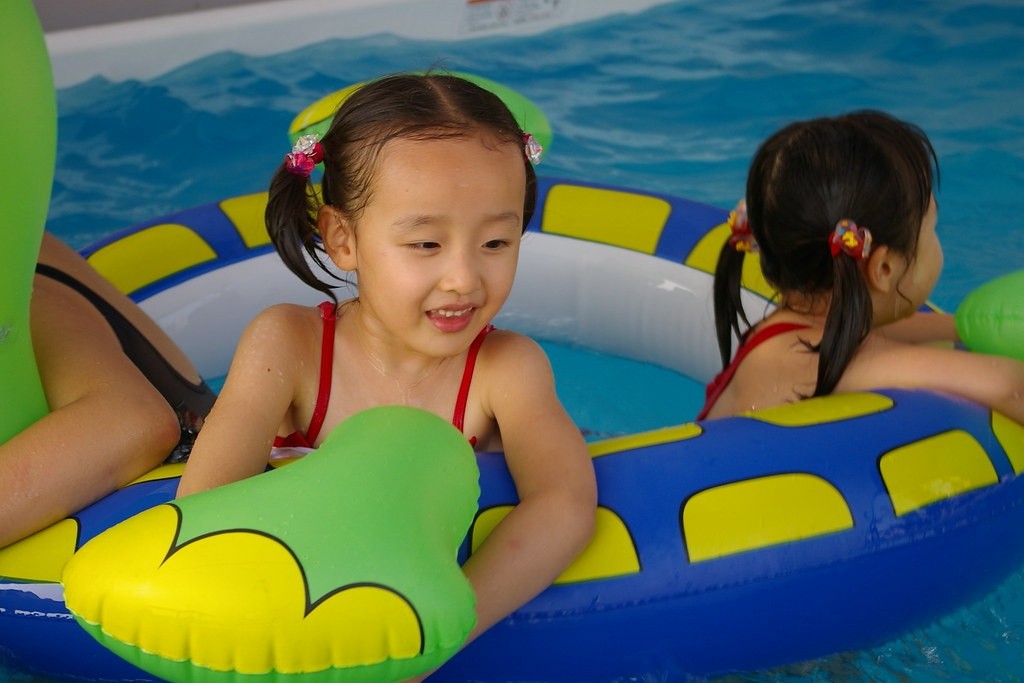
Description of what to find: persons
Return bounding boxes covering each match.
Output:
[175,74,597,683]
[695,111,1023,425]
[0,230,221,546]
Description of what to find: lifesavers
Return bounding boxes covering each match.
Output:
[1,0,1024,681]
